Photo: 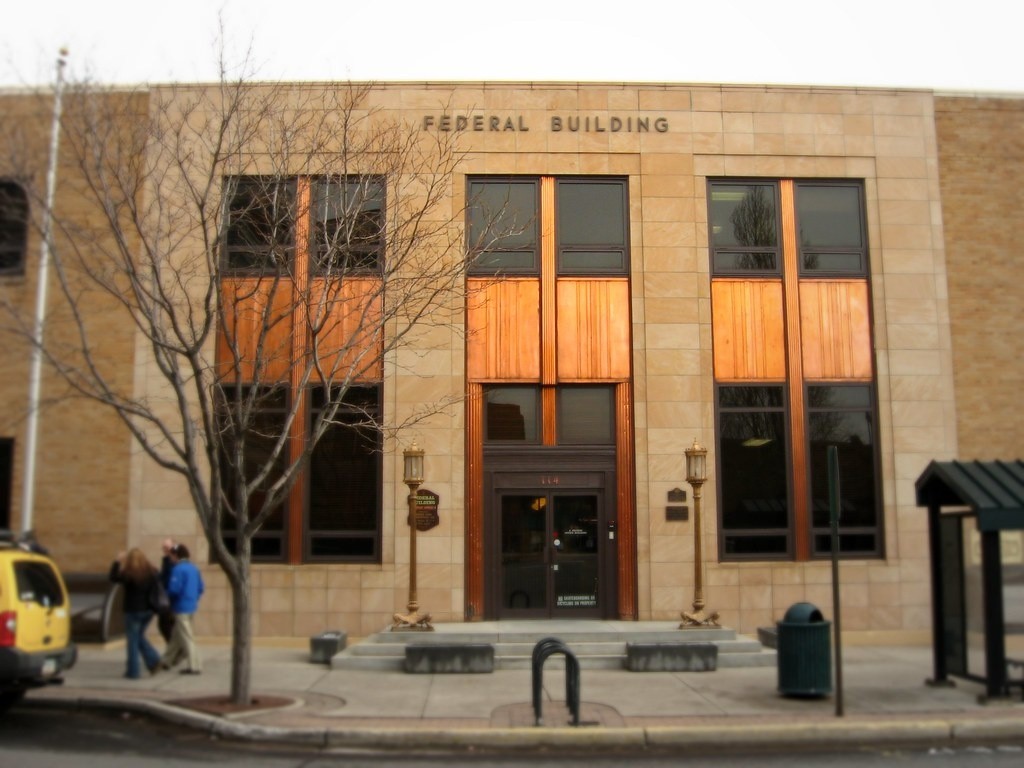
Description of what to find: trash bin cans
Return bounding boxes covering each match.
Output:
[777,602,833,702]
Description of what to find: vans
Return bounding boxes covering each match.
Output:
[1,530,78,717]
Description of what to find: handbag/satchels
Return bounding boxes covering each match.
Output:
[146,570,169,611]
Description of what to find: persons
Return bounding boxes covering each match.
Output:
[152,538,175,645]
[108,548,161,680]
[160,543,203,675]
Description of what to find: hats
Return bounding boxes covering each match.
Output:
[170,545,191,557]
